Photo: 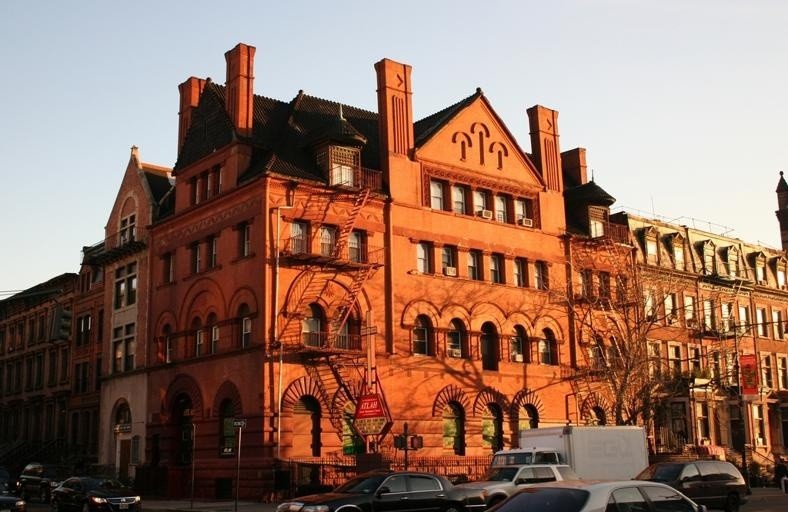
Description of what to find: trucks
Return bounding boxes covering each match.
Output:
[490,426,650,483]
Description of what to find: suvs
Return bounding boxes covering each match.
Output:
[0,467,25,496]
[20,462,71,503]
[632,460,749,511]
[454,464,581,511]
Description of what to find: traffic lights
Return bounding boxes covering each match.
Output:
[392,436,404,449]
[47,304,73,345]
[410,436,422,448]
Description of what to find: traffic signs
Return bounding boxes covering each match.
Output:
[233,419,246,427]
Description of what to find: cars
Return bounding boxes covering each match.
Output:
[0,481,26,512]
[51,476,143,512]
[483,479,708,511]
[276,471,454,511]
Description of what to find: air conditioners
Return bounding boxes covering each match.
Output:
[482,210,492,219]
[515,354,523,362]
[451,349,461,357]
[446,267,456,276]
[522,218,532,227]
